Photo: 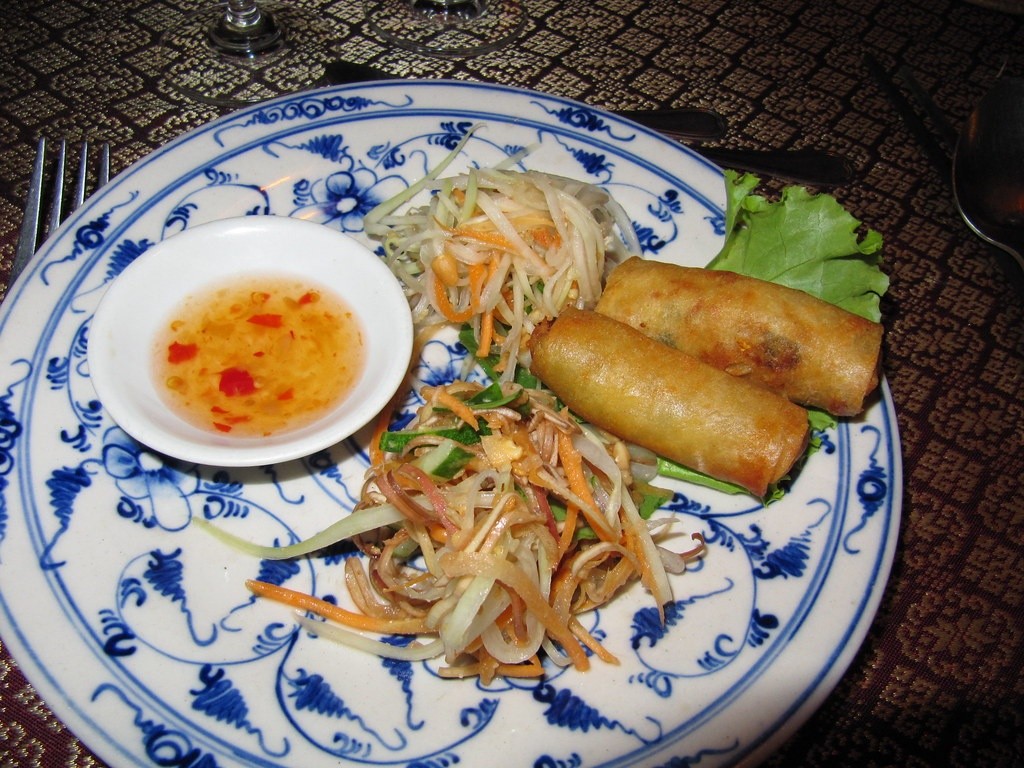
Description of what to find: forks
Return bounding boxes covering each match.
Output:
[5,138,109,291]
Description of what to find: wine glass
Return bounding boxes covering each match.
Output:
[203,1,287,60]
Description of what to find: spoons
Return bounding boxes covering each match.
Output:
[950,41,1024,278]
[328,59,731,143]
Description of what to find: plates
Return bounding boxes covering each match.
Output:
[0,77,900,767]
[86,216,413,467]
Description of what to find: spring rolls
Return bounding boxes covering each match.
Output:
[530,252,884,500]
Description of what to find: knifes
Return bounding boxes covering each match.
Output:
[163,83,865,193]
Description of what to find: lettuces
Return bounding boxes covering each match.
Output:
[654,167,892,500]
